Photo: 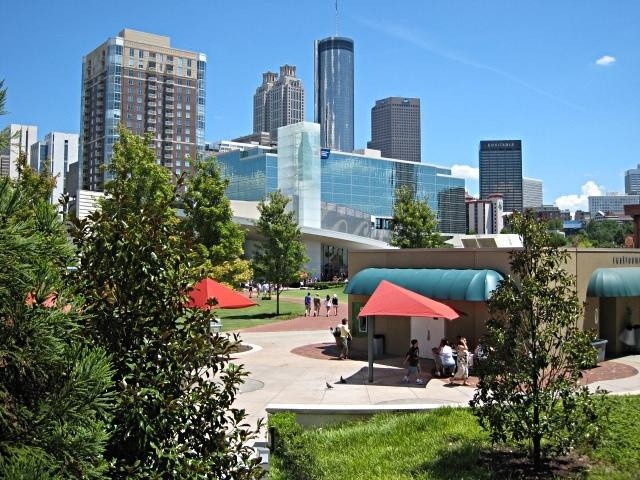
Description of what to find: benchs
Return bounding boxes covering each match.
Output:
[189,316,222,333]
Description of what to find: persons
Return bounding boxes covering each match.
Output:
[337,318,353,360]
[248,281,270,298]
[300,280,338,317]
[402,335,495,386]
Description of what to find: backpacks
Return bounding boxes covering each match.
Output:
[405,351,418,363]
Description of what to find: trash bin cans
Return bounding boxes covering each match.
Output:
[590,339,608,362]
[372,335,383,359]
[209,318,223,332]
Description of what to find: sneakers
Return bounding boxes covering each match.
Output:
[415,378,426,385]
[401,375,411,384]
